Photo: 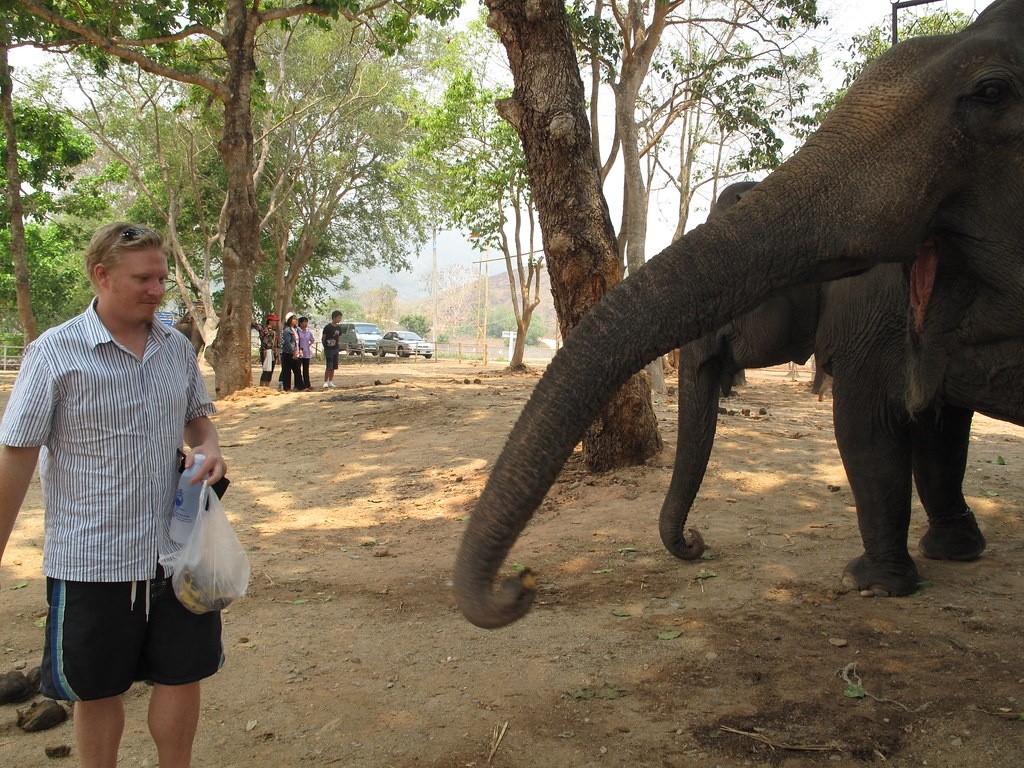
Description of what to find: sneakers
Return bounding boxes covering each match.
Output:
[323,383,329,388]
[328,383,336,388]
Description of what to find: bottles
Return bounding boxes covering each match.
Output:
[169,454,208,545]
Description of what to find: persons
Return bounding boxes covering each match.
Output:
[0,219,229,768]
[321,310,343,388]
[276,311,304,393]
[294,316,315,391]
[258,314,280,386]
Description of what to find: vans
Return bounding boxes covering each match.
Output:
[337,322,382,356]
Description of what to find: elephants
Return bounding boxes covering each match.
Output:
[449,1,1023,628]
[658,179,988,600]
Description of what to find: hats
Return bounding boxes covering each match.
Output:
[285,312,295,323]
[266,315,280,321]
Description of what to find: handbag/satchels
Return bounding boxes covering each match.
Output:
[158,481,251,615]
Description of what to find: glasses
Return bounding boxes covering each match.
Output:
[118,228,147,242]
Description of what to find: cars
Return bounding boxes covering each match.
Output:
[376,331,434,360]
